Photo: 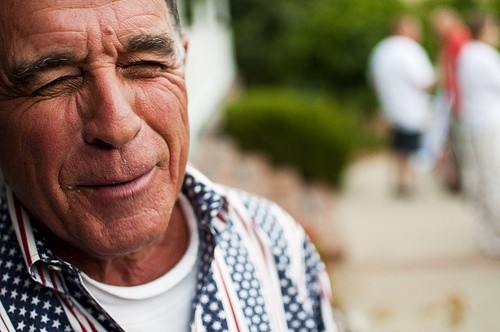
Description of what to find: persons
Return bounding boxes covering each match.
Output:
[367,1,500,259]
[0,0,336,331]
